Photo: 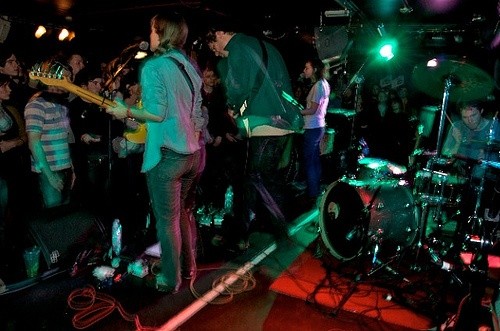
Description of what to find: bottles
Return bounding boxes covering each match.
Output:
[224,186,234,215]
[111,220,121,255]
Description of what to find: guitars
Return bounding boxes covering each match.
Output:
[28,61,148,143]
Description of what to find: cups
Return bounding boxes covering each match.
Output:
[24,246,39,276]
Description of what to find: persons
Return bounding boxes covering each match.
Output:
[0,52,225,205]
[24,65,75,208]
[442,101,500,176]
[207,29,305,250]
[300,61,331,195]
[344,78,411,162]
[106,15,203,293]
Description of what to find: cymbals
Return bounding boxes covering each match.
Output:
[461,137,500,150]
[357,158,407,175]
[412,58,494,101]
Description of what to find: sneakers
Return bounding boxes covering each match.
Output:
[148,272,179,293]
[181,268,197,279]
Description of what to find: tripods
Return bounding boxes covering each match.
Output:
[367,79,497,284]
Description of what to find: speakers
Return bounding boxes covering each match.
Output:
[21,212,109,279]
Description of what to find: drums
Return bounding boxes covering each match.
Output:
[318,176,419,262]
[413,171,466,206]
[469,158,500,189]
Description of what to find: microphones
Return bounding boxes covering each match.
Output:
[122,41,148,55]
[296,73,305,100]
[344,55,372,95]
[193,35,215,45]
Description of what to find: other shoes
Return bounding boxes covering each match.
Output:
[471,250,488,270]
[300,196,315,203]
